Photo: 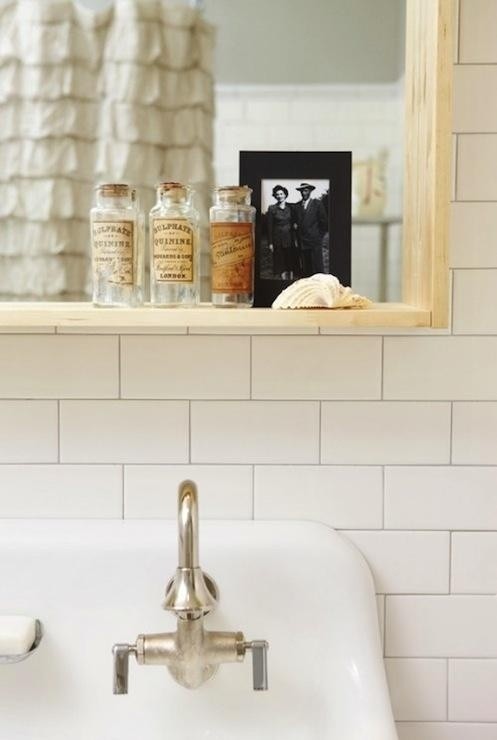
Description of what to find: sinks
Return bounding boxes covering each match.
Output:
[2,518,399,739]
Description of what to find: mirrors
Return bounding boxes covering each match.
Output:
[0,0,453,328]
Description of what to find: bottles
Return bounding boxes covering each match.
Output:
[144,182,200,304]
[206,184,257,310]
[86,182,147,307]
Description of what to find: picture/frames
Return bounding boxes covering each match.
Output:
[237,152,352,307]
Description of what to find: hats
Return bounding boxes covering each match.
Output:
[296,183,315,191]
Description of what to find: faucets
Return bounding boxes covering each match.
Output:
[111,481,268,692]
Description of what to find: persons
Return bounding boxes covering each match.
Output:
[261,184,295,279]
[288,182,330,280]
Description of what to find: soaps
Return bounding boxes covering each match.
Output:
[0,616,40,656]
